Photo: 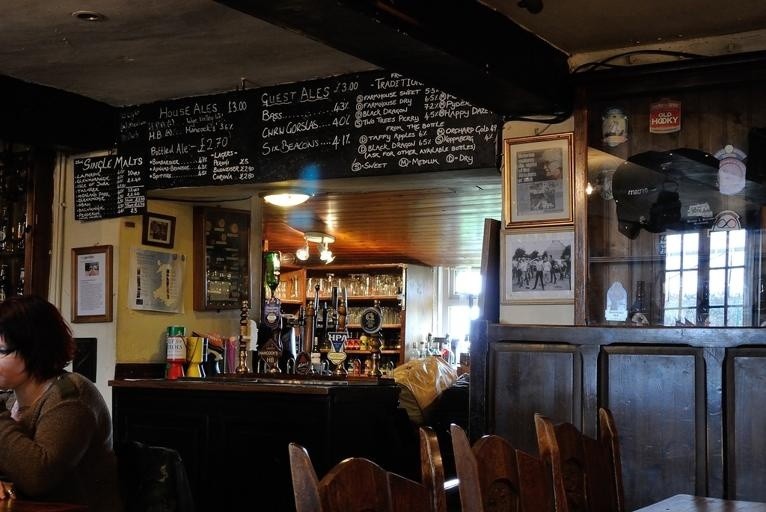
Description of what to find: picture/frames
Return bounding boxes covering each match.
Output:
[71,245,113,324]
[499,131,575,306]
[141,211,176,249]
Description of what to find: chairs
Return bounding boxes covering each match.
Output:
[120,440,182,512]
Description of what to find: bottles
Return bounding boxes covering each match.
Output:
[696,281,709,325]
[264,251,280,299]
[459,352,469,374]
[311,336,321,364]
[408,334,455,366]
[630,280,649,328]
[346,331,367,350]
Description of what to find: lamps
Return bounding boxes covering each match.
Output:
[296,231,336,265]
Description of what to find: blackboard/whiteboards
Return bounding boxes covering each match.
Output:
[116,66,505,192]
[74,152,147,221]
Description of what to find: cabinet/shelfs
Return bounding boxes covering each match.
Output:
[265,263,434,377]
[193,205,251,311]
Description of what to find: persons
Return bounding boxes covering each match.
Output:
[530,184,556,211]
[537,149,563,180]
[512,251,572,291]
[0,293,120,509]
